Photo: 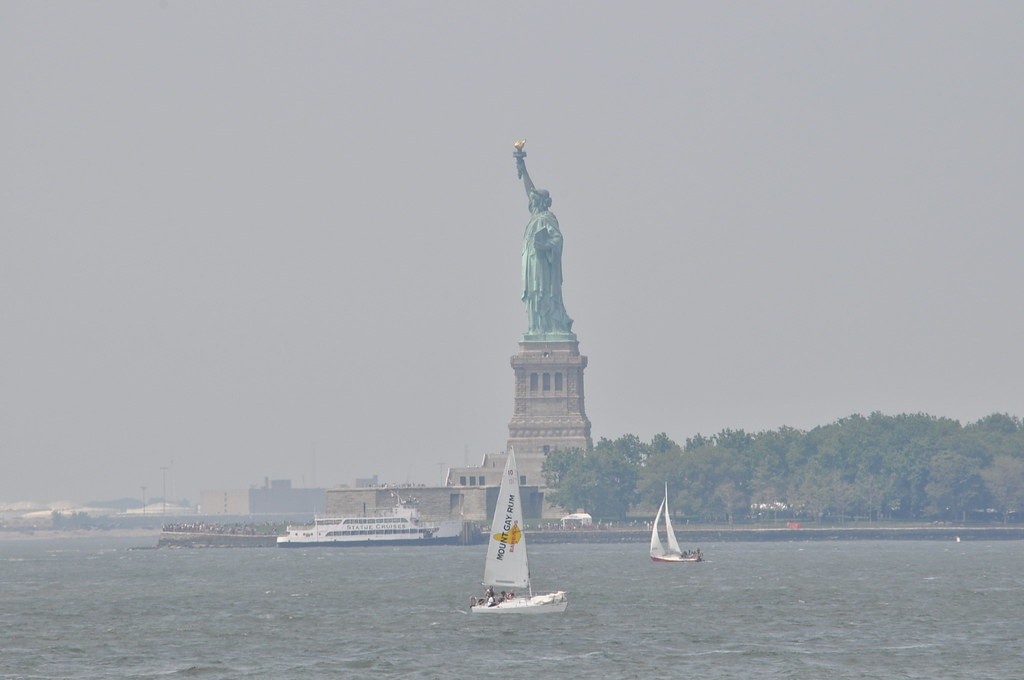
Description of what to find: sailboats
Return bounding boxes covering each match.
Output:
[649,480,704,562]
[467,444,568,616]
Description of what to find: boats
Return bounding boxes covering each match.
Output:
[275,500,460,547]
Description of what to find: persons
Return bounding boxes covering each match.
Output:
[681,548,702,560]
[477,586,515,607]
[512,140,573,334]
